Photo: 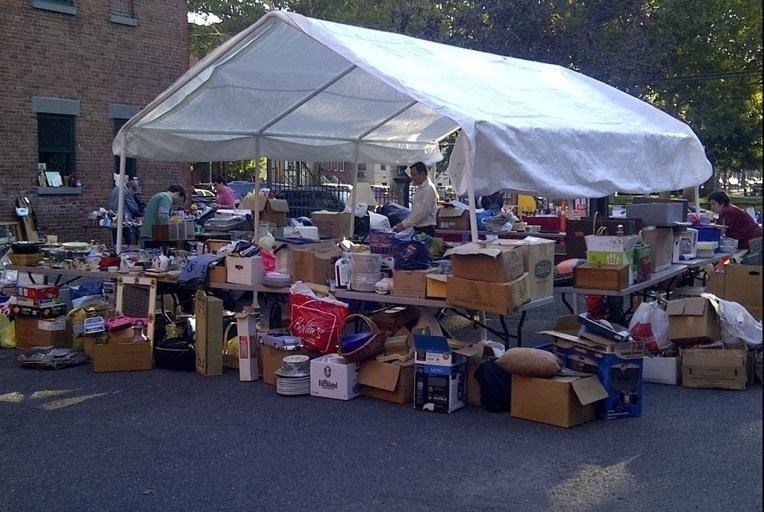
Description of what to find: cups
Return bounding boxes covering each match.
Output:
[106,266,118,273]
[120,246,192,271]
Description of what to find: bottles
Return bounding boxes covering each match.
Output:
[130,326,147,342]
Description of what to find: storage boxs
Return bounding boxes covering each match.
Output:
[2,185,763,429]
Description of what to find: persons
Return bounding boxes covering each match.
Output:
[138,185,185,249]
[709,191,763,254]
[108,181,139,244]
[392,162,440,237]
[209,176,236,209]
[481,191,503,240]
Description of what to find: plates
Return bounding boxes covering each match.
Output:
[274,369,310,396]
[264,270,292,288]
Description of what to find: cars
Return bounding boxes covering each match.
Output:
[193,181,454,222]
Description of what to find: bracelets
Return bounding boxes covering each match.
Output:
[218,203,220,209]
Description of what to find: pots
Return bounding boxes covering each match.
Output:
[609,363,641,391]
[324,365,331,378]
[8,239,46,267]
[566,353,598,373]
